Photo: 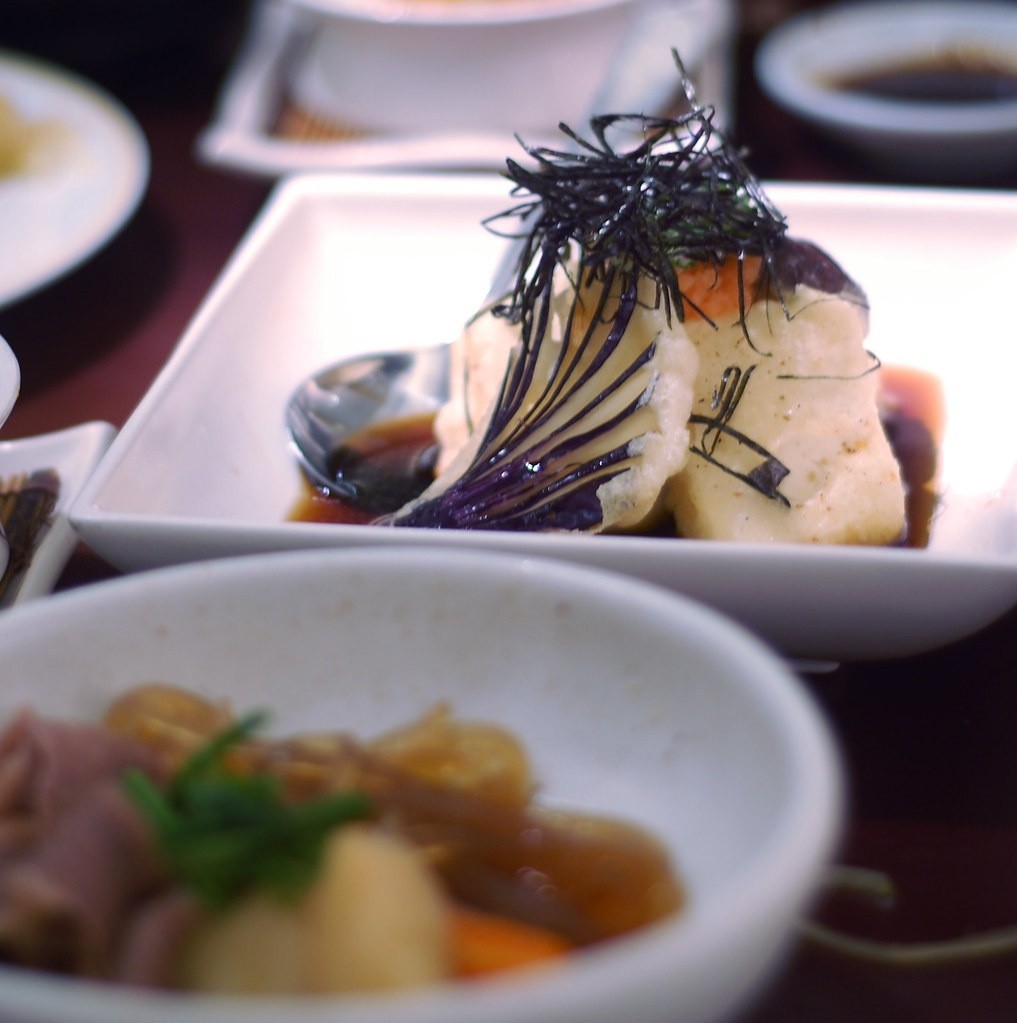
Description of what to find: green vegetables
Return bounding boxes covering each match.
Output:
[117,694,378,903]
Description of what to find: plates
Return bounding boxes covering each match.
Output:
[62,169,1017,680]
[0,48,152,311]
[192,0,737,180]
[0,421,116,616]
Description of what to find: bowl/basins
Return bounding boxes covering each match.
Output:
[3,545,847,1023]
[754,1,1017,180]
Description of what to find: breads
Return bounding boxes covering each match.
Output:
[431,255,697,528]
[665,285,905,545]
